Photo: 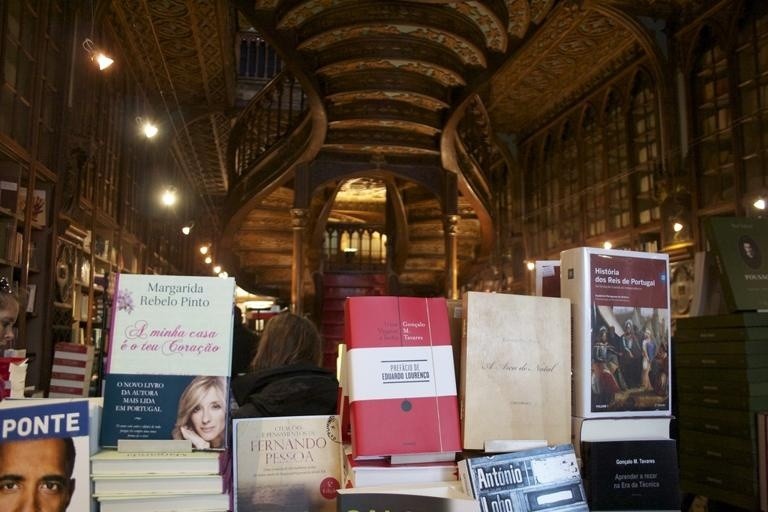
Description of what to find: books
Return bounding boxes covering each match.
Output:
[1,272,347,509]
[337,248,690,510]
[670,215,768,510]
[2,175,124,350]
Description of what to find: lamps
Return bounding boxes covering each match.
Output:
[134,116,158,138]
[82,38,114,71]
[181,219,197,236]
